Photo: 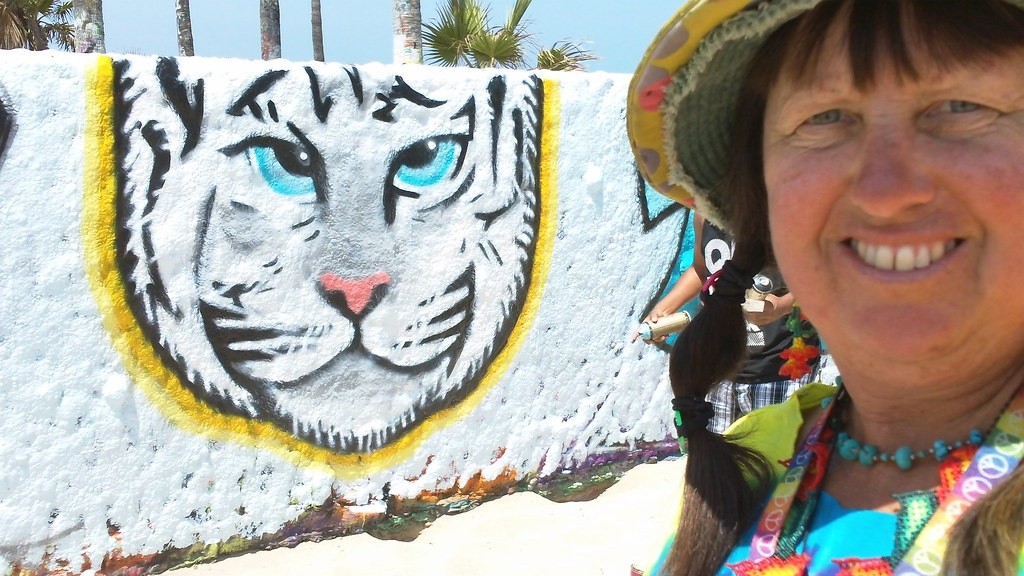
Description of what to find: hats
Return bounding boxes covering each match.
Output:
[626,0,821,239]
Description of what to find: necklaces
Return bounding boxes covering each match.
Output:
[828,375,1024,472]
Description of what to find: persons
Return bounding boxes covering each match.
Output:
[644,0,1024,576]
[629,210,828,457]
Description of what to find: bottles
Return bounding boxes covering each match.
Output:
[637,310,692,341]
[745,274,773,301]
